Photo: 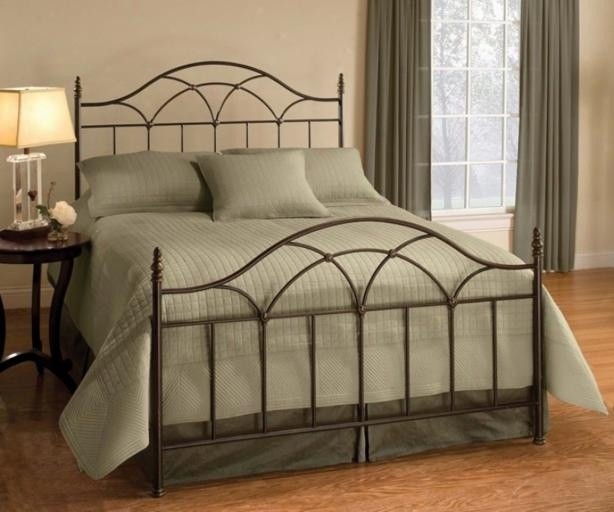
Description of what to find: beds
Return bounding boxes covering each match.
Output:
[72,61,545,498]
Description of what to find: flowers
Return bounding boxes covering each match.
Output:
[34,185,77,237]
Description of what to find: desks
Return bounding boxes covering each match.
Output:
[1,228,90,390]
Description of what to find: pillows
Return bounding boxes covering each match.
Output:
[194,149,331,223]
[220,147,387,205]
[79,150,220,218]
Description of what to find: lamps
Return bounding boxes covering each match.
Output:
[0,86,79,240]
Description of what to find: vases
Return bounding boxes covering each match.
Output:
[48,224,68,242]
